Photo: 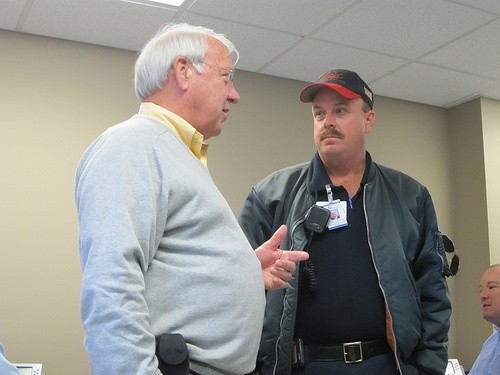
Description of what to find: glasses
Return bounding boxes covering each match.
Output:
[201,62,233,81]
[441,234,460,276]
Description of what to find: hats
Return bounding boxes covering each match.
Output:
[300,69,374,109]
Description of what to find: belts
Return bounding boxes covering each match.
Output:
[298,339,390,364]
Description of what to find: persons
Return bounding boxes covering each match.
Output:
[331,211,340,220]
[237,69,452,375]
[467,264,500,375]
[73,23,310,375]
